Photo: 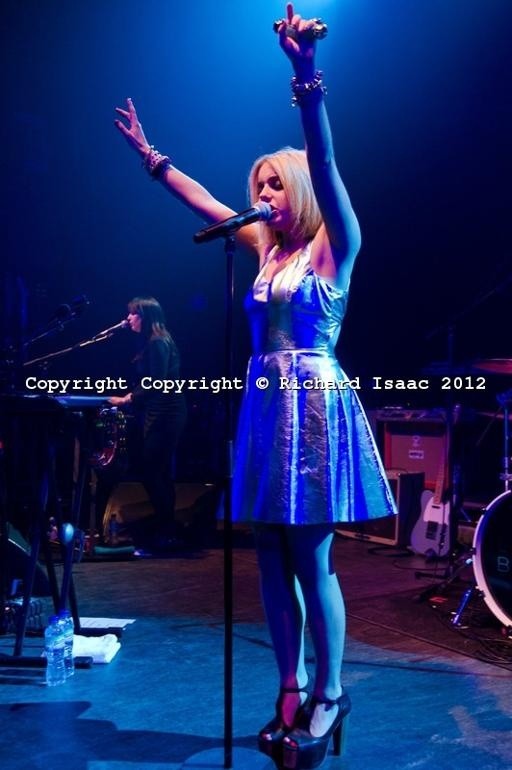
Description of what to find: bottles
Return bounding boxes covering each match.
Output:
[107,514,118,546]
[44,608,75,688]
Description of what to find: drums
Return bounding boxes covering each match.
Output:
[470,488,512,632]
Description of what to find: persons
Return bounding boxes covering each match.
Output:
[110,1,404,770]
[106,292,190,563]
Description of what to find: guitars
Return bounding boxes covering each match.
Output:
[409,401,460,562]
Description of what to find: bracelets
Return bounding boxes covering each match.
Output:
[290,88,328,110]
[141,145,173,177]
[290,70,323,94]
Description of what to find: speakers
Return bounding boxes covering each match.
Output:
[334,470,425,547]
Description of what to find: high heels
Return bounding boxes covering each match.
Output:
[257,673,353,770]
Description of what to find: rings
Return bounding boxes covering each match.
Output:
[312,16,324,25]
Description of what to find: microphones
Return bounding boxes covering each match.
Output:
[107,320,128,332]
[193,201,271,244]
